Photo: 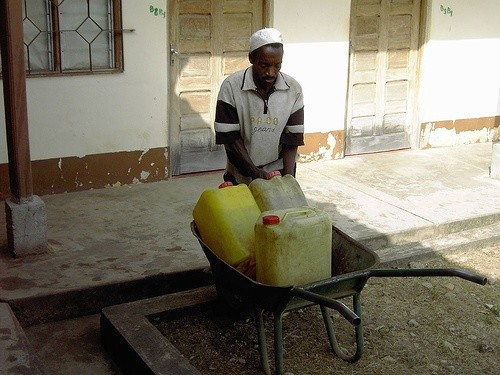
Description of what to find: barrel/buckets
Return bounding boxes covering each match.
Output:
[247,171,308,212]
[253,206,333,287]
[192,182,262,265]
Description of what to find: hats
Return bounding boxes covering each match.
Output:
[249,26,283,54]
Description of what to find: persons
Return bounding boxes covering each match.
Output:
[212,27,306,187]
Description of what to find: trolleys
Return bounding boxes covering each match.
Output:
[190,217,487,375]
